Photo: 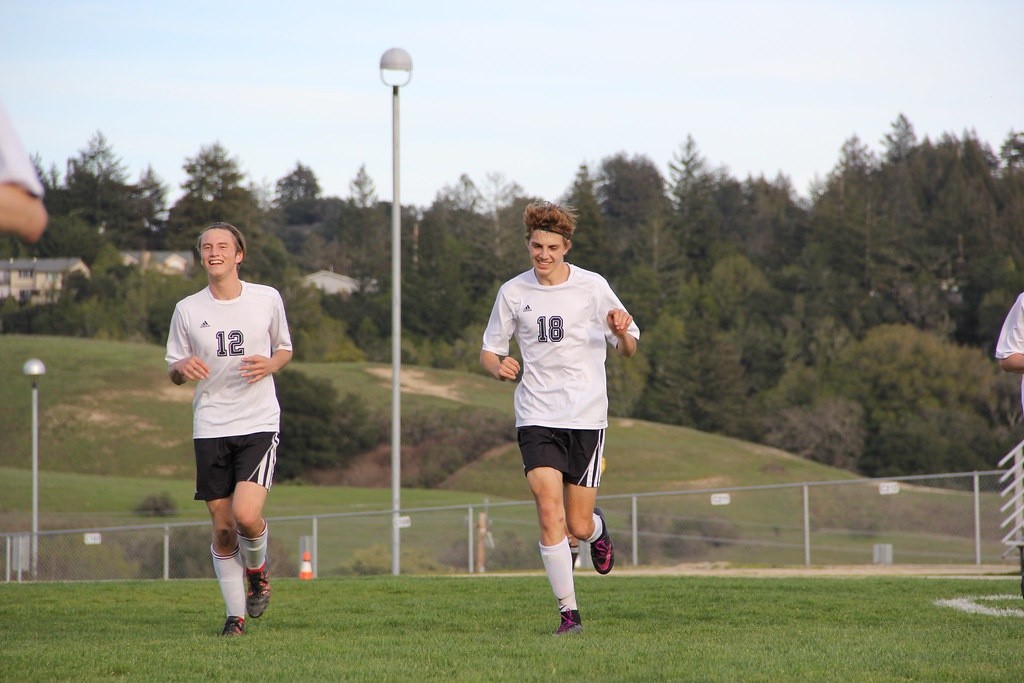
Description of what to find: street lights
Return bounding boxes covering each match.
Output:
[378,48,414,576]
[22,359,46,580]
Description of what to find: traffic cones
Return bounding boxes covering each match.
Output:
[299,552,313,580]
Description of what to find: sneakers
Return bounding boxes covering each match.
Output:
[222,615,246,637]
[591,507,615,574]
[245,560,270,618]
[554,607,583,637]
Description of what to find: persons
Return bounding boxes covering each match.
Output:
[995,292,1024,410]
[480,201,640,637]
[164,222,293,637]
[0,104,49,244]
